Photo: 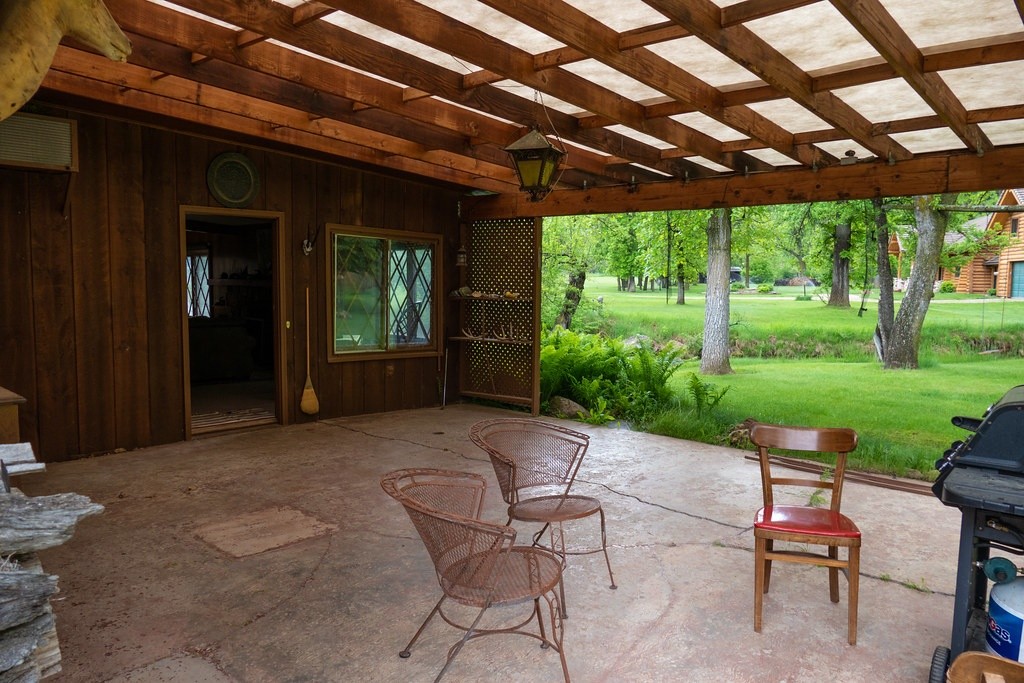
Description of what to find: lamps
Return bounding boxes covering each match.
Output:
[456,245,469,268]
[501,89,569,206]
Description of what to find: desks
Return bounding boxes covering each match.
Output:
[0,386,29,490]
[192,320,252,386]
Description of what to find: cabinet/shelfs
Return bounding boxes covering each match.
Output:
[448,296,534,345]
[929,387,1024,683]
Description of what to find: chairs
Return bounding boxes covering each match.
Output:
[749,423,862,645]
[464,418,618,621]
[381,467,571,683]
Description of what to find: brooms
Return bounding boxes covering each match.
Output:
[300,287,320,414]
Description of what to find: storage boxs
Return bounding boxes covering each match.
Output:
[947,651,1024,683]
